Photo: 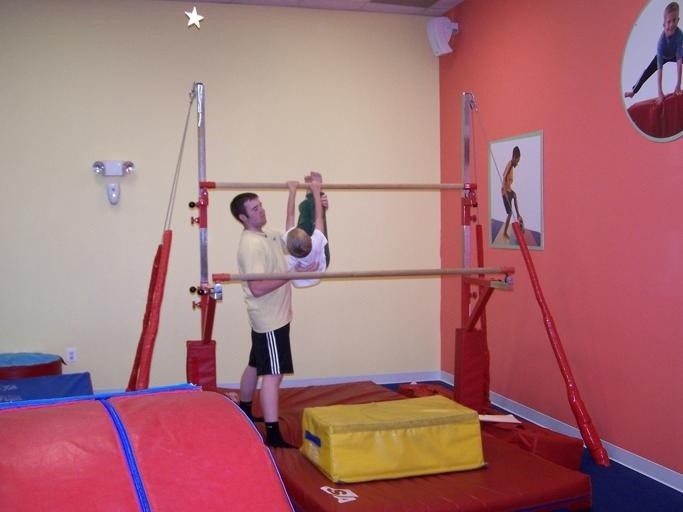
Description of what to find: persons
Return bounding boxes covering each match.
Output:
[281,172,330,289]
[624,2,682,99]
[500,146,526,239]
[230,193,327,447]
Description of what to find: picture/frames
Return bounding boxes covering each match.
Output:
[618,0,683,143]
[486,129,545,251]
